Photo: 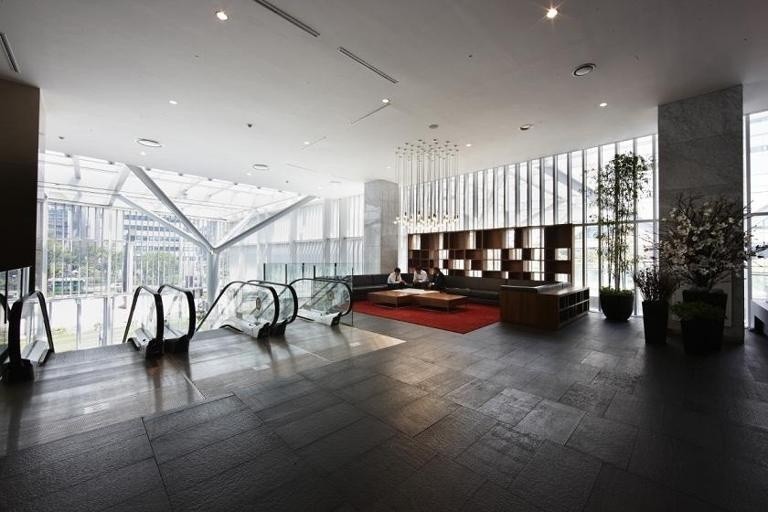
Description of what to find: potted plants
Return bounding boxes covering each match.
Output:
[583,152,680,355]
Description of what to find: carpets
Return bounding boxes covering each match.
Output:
[351,299,501,335]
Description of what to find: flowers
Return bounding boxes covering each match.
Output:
[646,190,767,290]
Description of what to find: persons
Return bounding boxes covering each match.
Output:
[386,267,408,289]
[428,266,447,290]
[412,264,430,289]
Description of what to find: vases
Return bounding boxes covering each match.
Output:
[681,286,728,349]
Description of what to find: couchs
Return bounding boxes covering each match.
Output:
[351,274,571,299]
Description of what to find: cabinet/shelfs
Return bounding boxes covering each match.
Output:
[528,286,590,329]
[408,223,575,285]
